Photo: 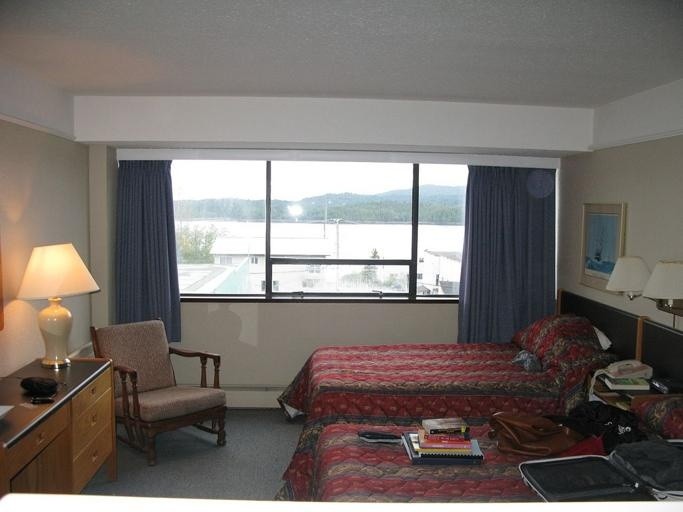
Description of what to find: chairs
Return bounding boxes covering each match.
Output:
[88,318,229,467]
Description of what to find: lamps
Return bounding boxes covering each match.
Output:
[15,241,103,370]
[605,255,683,312]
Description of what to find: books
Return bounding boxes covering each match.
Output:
[602,376,650,392]
[358,430,401,444]
[401,416,486,467]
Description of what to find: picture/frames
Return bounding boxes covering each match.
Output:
[576,201,627,296]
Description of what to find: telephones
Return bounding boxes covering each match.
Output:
[605,360,653,380]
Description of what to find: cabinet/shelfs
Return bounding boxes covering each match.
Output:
[0,356,119,501]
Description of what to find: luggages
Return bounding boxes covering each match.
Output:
[518,438,682,501]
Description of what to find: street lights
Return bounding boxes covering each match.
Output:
[333,216,346,271]
